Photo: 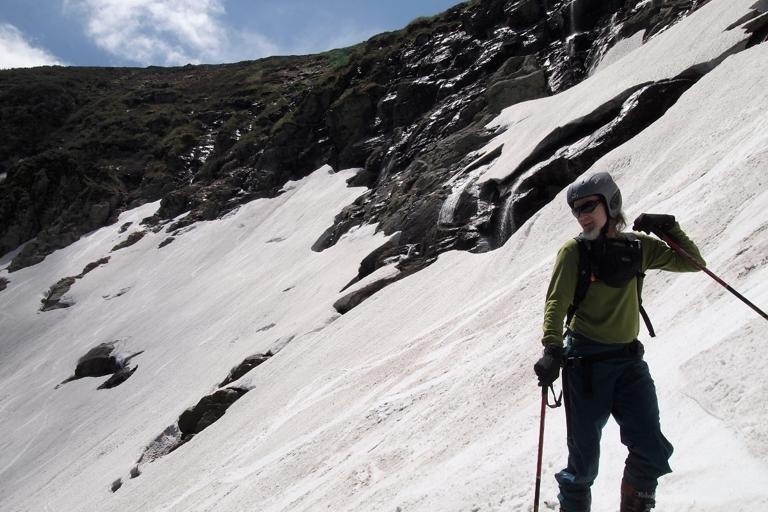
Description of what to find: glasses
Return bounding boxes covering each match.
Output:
[569,201,606,218]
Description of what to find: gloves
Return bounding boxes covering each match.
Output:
[632,212,676,236]
[533,345,565,387]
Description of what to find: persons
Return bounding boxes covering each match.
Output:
[535,171,706,511]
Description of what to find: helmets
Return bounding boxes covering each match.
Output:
[565,172,623,219]
